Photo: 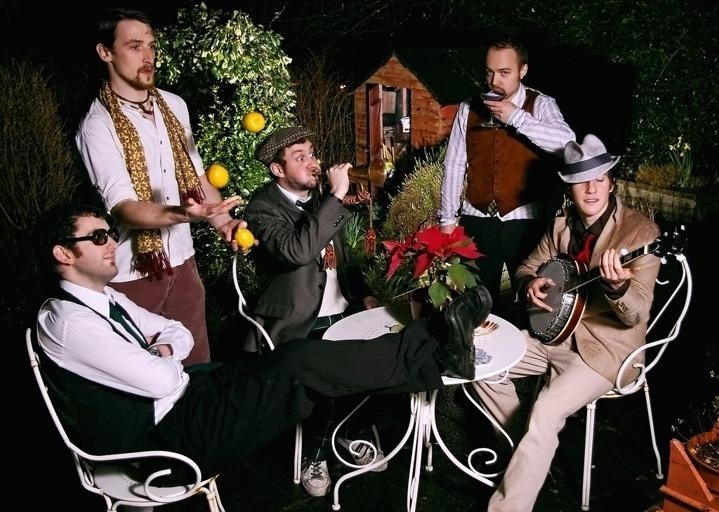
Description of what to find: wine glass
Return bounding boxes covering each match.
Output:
[479,92,507,128]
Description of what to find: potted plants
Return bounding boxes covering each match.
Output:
[671,369,719,493]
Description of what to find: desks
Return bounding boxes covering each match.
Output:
[322,304,526,512]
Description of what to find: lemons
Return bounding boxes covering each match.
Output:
[206,162,231,187]
[243,111,266,134]
[234,227,254,249]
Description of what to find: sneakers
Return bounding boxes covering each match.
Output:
[300,455,332,498]
[336,437,390,473]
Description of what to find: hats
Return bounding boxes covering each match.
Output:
[253,126,317,167]
[552,134,623,186]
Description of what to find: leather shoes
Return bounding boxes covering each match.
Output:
[439,299,476,382]
[454,284,494,329]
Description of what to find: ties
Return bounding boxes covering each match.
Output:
[109,303,148,350]
[578,234,596,262]
[294,199,316,214]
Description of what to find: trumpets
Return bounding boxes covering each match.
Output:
[312,159,387,203]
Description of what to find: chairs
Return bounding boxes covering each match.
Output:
[232,253,385,485]
[26,327,224,512]
[528,247,693,511]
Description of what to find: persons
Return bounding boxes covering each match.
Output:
[30,199,496,489]
[467,132,665,512]
[245,126,390,498]
[437,32,577,405]
[73,7,260,367]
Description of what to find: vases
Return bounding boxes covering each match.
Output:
[409,299,436,320]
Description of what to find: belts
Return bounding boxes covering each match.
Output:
[314,310,350,331]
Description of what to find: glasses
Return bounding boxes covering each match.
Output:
[62,226,121,247]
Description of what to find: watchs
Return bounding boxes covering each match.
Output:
[145,347,162,358]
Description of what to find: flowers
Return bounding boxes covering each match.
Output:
[381,224,487,312]
